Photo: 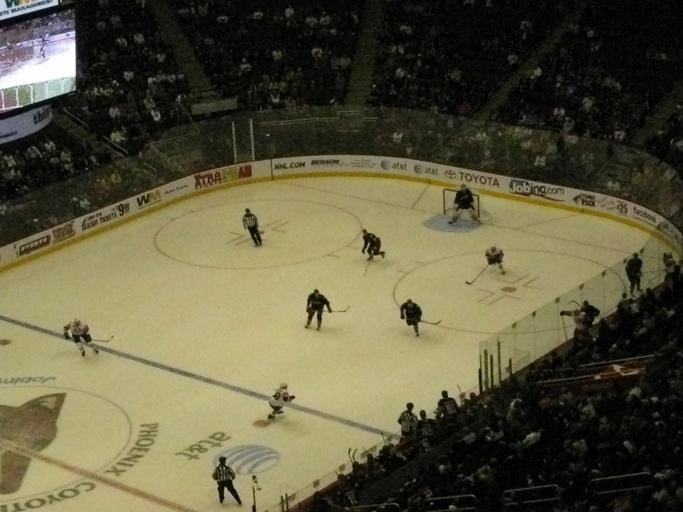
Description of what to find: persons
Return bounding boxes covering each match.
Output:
[212,456,244,506]
[483,244,507,277]
[0,2,683,241]
[267,383,297,419]
[483,250,681,511]
[304,288,333,331]
[60,316,98,356]
[309,390,485,511]
[242,207,265,246]
[361,229,385,259]
[399,299,422,335]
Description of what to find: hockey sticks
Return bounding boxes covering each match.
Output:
[465,264,489,284]
[90,336,114,342]
[421,320,441,325]
[322,305,350,312]
